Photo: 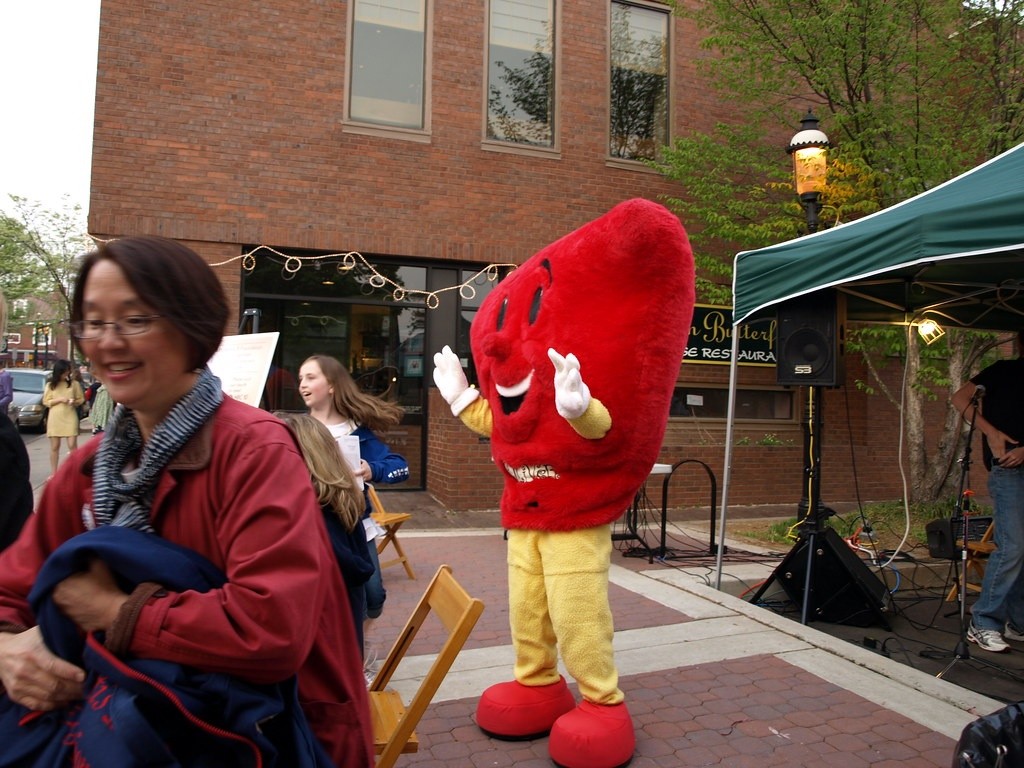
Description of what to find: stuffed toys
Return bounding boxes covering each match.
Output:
[432,197,695,768]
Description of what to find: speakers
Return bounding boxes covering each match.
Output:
[775,287,847,390]
[773,526,891,627]
[926,517,994,561]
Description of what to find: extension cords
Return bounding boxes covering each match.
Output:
[847,640,889,657]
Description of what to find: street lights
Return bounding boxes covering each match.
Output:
[43,324,51,368]
[784,107,833,536]
[32,312,42,368]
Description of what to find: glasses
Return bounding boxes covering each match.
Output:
[67,314,167,340]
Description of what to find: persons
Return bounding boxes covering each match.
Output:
[42,359,84,482]
[71,369,121,437]
[952,328,1024,653]
[0,234,373,768]
[273,356,411,664]
[0,361,13,415]
[0,411,33,551]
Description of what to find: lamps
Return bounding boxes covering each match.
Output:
[915,315,947,346]
[320,256,339,286]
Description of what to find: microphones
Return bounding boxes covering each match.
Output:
[969,385,986,403]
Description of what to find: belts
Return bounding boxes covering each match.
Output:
[992,458,1006,466]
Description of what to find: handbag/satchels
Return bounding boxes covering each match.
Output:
[951,702,1024,768]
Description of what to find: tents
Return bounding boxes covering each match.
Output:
[713,141,1024,592]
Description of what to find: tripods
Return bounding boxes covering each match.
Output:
[921,399,1024,683]
[749,387,894,632]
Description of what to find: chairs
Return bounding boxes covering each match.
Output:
[946,520,999,602]
[366,564,485,768]
[365,481,418,580]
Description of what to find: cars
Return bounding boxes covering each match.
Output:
[3,368,54,434]
[76,371,98,420]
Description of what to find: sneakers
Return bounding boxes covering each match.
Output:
[966,619,1011,652]
[1005,620,1024,641]
